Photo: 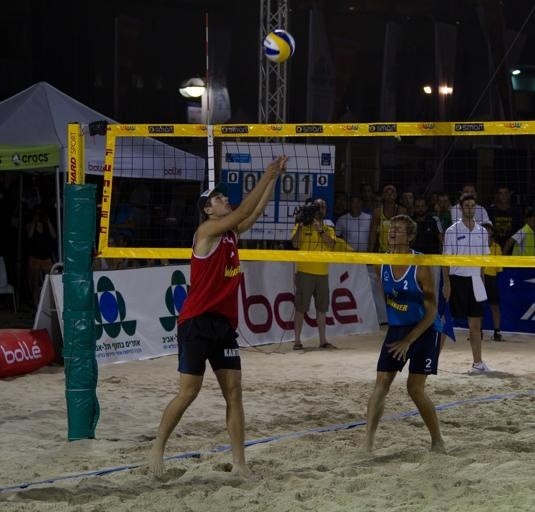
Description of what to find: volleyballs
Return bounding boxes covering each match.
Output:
[264,30,296,62]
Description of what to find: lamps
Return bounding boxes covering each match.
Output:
[177,76,206,98]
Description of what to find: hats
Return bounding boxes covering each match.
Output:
[198,182,228,210]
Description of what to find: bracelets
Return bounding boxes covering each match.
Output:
[319,230,324,234]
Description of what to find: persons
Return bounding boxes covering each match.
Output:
[151,155,292,478]
[439,193,491,375]
[357,213,448,457]
[289,197,338,351]
[334,179,535,266]
[27,214,57,316]
[465,222,503,342]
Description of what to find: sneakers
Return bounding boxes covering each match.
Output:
[320,343,336,349]
[472,362,497,373]
[293,344,302,350]
